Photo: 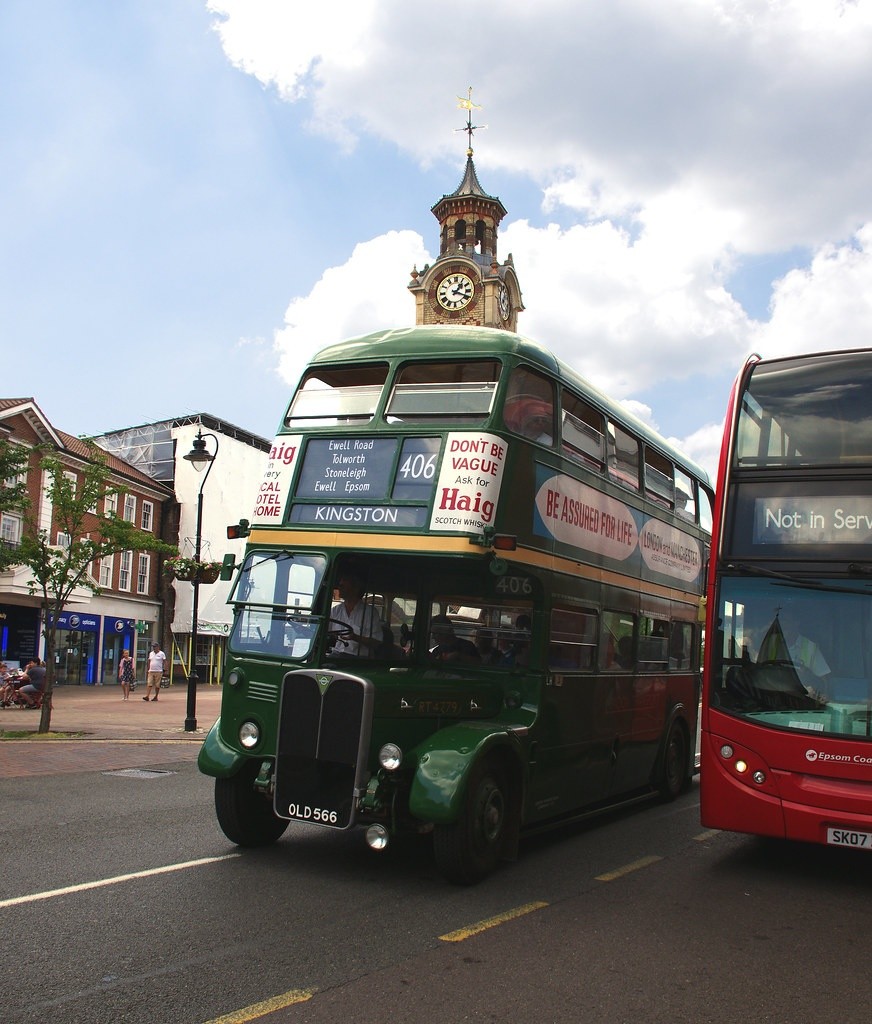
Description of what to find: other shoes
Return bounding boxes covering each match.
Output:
[151,697,158,701]
[29,703,37,709]
[143,696,149,701]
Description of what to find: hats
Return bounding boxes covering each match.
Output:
[151,642,159,646]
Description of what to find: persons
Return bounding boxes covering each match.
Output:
[118,649,135,702]
[290,566,832,705]
[0,656,50,709]
[142,642,168,701]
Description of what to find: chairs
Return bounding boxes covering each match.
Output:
[32,674,54,710]
[2,679,30,709]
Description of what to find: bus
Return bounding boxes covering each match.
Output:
[198,326,715,889]
[698,346,872,851]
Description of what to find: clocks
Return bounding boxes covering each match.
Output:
[498,282,511,322]
[436,273,475,311]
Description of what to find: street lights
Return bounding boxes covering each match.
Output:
[183,427,219,732]
